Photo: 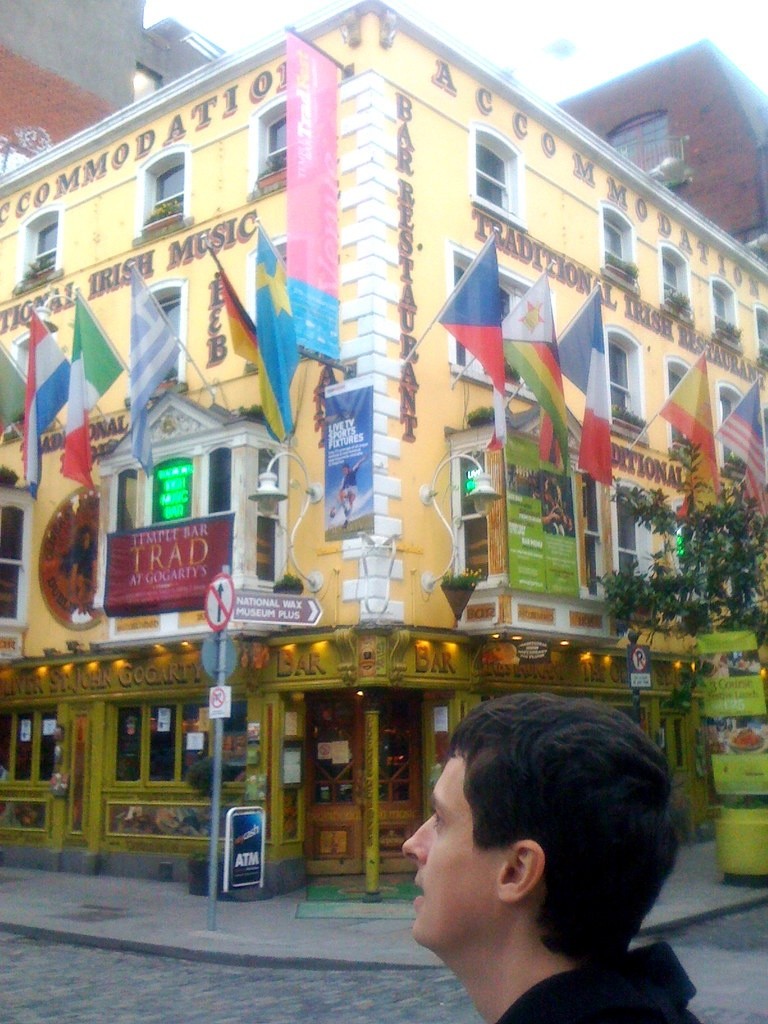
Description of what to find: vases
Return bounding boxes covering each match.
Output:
[141,212,183,237]
[22,265,54,286]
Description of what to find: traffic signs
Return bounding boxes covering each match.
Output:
[230,590,323,626]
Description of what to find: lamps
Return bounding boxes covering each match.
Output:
[23,294,75,334]
[248,452,324,593]
[417,454,503,594]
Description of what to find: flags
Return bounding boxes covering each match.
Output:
[256,230,300,442]
[0,344,28,437]
[438,238,507,450]
[502,274,567,469]
[130,270,181,478]
[219,274,258,366]
[23,312,71,500]
[715,380,768,518]
[661,351,718,518]
[62,296,125,491]
[559,292,612,485]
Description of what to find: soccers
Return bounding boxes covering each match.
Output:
[328,525,336,533]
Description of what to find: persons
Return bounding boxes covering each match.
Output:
[338,454,368,528]
[541,475,572,531]
[402,694,703,1024]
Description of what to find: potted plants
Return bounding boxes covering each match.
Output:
[604,252,639,288]
[255,154,287,189]
[716,318,742,346]
[665,290,692,320]
[611,404,647,434]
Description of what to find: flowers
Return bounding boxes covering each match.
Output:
[21,261,55,279]
[271,572,304,594]
[143,198,181,225]
[440,567,482,589]
